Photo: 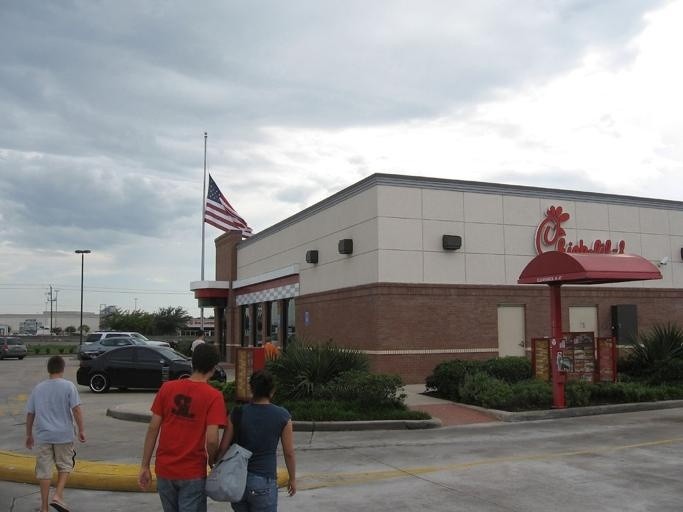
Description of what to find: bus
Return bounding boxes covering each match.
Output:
[24,318,38,336]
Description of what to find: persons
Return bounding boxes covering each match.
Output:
[138,343,228,512]
[25,355,86,512]
[191,331,206,353]
[216,370,297,512]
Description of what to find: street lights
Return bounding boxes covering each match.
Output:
[72,247,95,344]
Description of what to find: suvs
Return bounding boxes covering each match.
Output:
[85,331,172,350]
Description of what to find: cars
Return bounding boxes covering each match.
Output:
[0,337,28,360]
[10,331,35,337]
[77,343,228,392]
[76,336,151,359]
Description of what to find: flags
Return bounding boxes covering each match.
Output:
[205,173,253,238]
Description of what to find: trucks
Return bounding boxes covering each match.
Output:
[0,324,10,336]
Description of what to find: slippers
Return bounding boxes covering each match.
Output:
[49,498,73,512]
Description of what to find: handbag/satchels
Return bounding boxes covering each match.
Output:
[204,442,254,503]
